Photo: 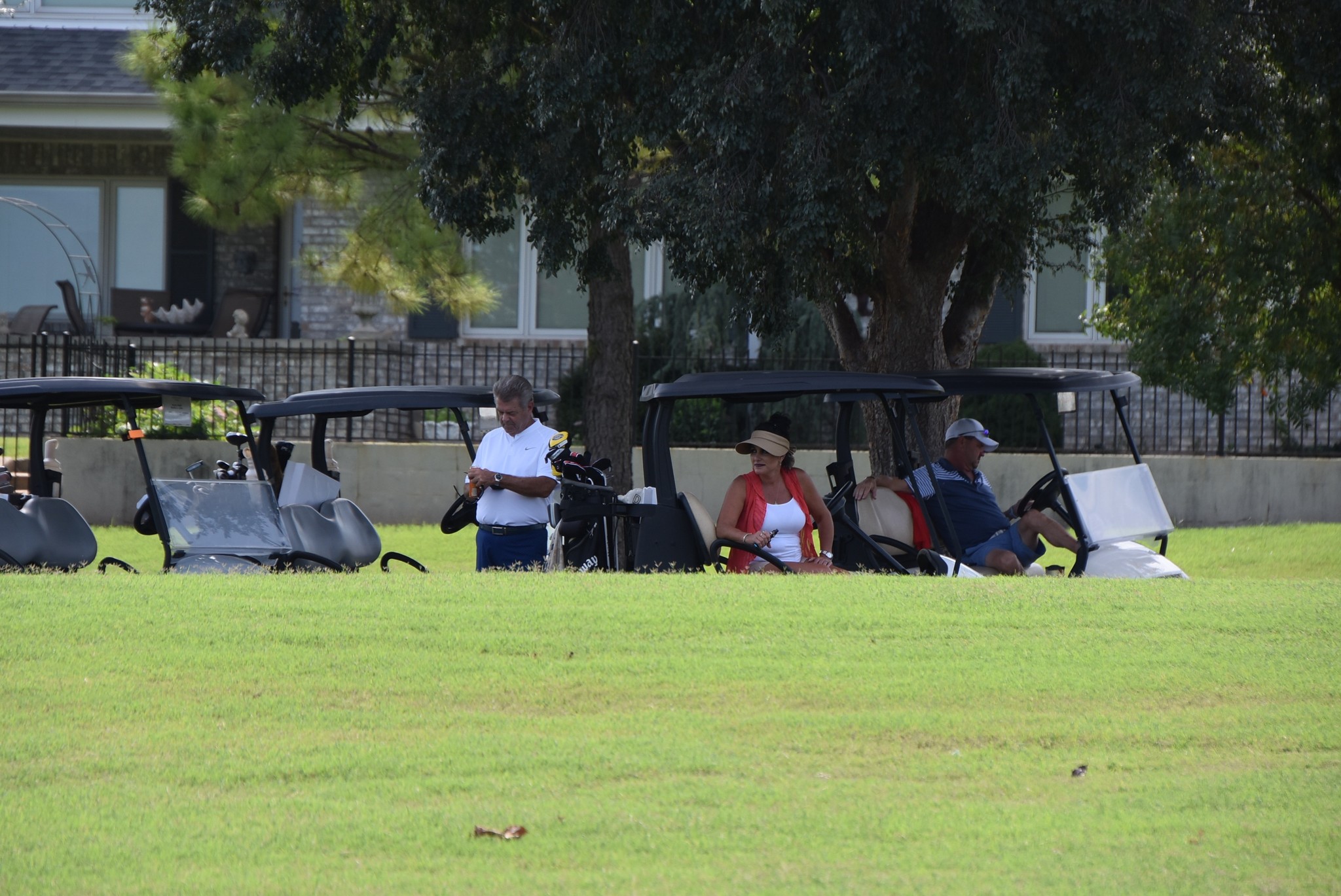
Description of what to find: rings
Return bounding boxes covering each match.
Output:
[760,533,763,536]
[762,531,765,533]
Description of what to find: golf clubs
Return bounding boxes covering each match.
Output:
[0,466,15,494]
[584,456,616,486]
[213,431,248,481]
[185,459,203,480]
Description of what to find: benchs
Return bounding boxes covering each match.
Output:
[278,498,429,575]
[853,486,1046,578]
[0,496,139,574]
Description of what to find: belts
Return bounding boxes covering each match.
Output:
[480,523,547,536]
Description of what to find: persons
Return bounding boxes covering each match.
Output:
[716,411,847,574]
[852,418,1082,576]
[464,375,564,573]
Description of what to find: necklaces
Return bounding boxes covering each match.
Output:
[763,479,780,505]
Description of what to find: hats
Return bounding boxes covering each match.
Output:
[945,418,999,452]
[735,430,796,456]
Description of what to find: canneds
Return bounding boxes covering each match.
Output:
[469,466,481,497]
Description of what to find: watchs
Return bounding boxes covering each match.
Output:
[819,550,833,559]
[494,472,503,486]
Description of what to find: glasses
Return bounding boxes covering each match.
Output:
[959,429,990,437]
[453,472,484,505]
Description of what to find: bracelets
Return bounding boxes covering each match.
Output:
[743,533,752,544]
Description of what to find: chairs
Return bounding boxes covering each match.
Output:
[677,490,798,573]
[7,279,272,373]
